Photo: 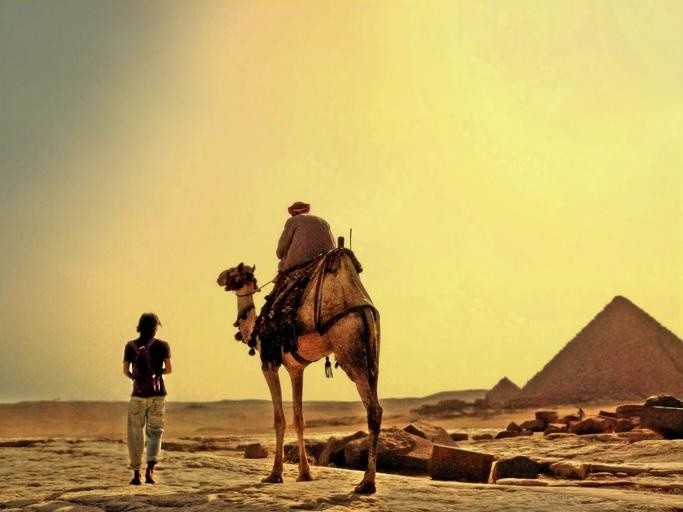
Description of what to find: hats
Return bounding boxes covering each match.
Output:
[138,313,162,330]
[288,202,310,215]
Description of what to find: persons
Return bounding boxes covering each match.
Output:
[122,311,173,487]
[275,201,337,278]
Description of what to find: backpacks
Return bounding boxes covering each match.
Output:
[128,339,160,397]
